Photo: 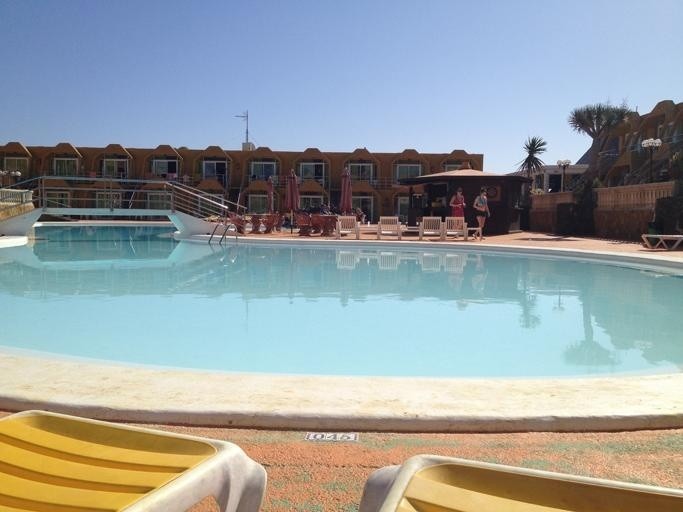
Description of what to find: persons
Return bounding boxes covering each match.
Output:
[319,203,331,215]
[447,256,468,309]
[471,189,490,240]
[447,186,466,239]
[353,207,364,223]
[470,254,489,304]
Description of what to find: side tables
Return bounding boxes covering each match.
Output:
[466,227,483,241]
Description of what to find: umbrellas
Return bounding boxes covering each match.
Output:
[284,266,297,304]
[339,166,353,216]
[282,166,300,234]
[265,173,274,215]
[337,269,350,308]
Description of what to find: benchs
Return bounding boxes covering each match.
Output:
[641,234,682,251]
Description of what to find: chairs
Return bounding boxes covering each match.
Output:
[337,216,359,240]
[445,217,468,241]
[0,412,267,512]
[359,453,683,512]
[376,216,401,240]
[336,251,463,273]
[419,216,444,240]
